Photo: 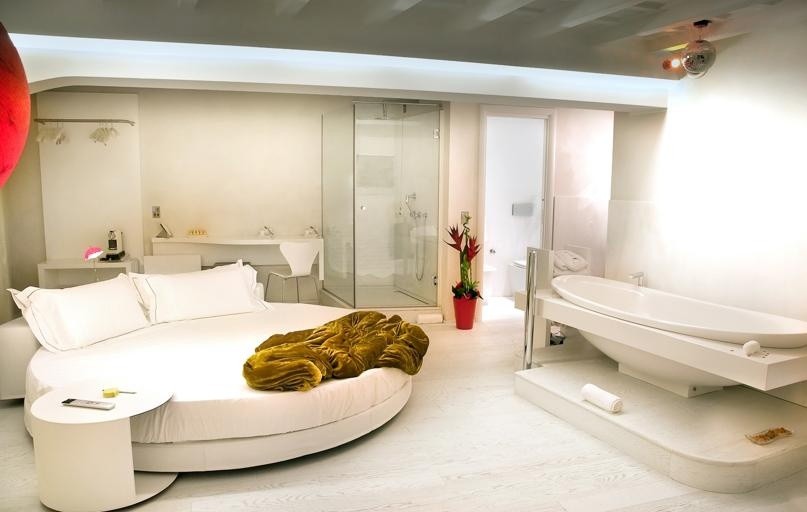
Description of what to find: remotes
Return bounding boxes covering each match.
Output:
[60,399,115,410]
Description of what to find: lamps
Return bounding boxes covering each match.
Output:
[682,18,717,75]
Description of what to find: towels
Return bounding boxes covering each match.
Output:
[578,382,623,413]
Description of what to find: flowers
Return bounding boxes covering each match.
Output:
[440,214,488,300]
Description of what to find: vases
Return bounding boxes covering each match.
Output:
[452,295,478,329]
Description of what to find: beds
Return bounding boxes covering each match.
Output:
[23,272,414,473]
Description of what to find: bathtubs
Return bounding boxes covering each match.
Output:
[550,272,807,387]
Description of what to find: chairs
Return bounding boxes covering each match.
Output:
[263,240,321,305]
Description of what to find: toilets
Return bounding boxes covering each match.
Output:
[508,258,528,296]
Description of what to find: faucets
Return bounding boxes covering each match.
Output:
[264,225,275,237]
[311,225,320,238]
[629,270,644,287]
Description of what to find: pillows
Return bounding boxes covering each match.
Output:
[5,257,272,355]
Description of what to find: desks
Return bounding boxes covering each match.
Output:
[29,374,178,512]
